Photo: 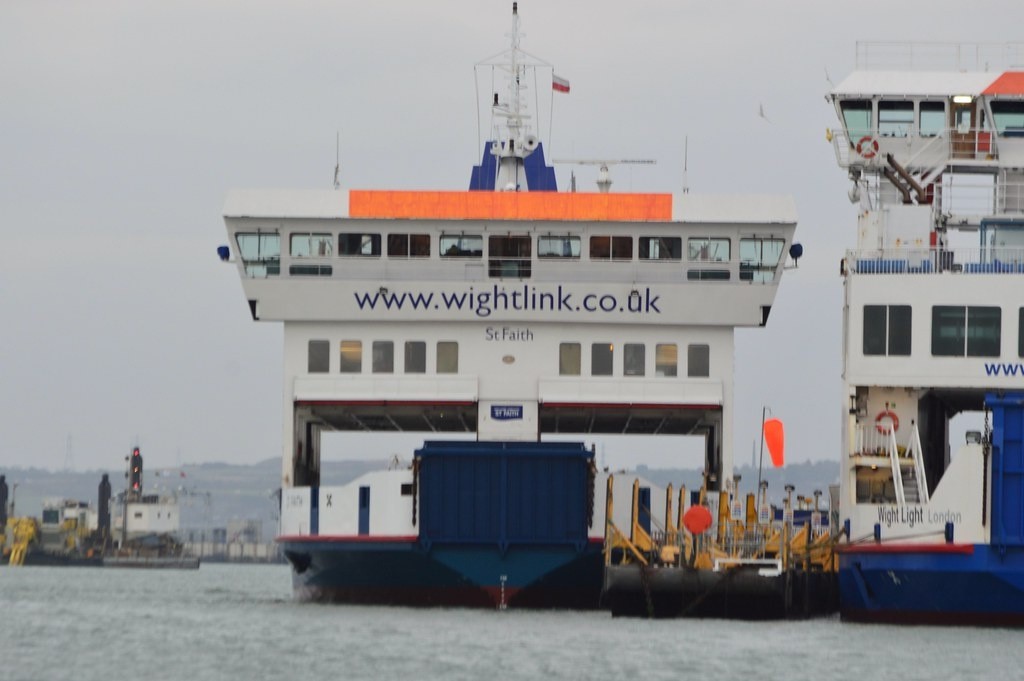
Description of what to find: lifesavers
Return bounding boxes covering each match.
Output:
[857,136,879,159]
[875,410,899,435]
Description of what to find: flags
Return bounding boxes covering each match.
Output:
[551,72,570,94]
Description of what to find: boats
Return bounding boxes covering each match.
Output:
[0,447,202,570]
[213,0,803,616]
[816,61,1024,629]
